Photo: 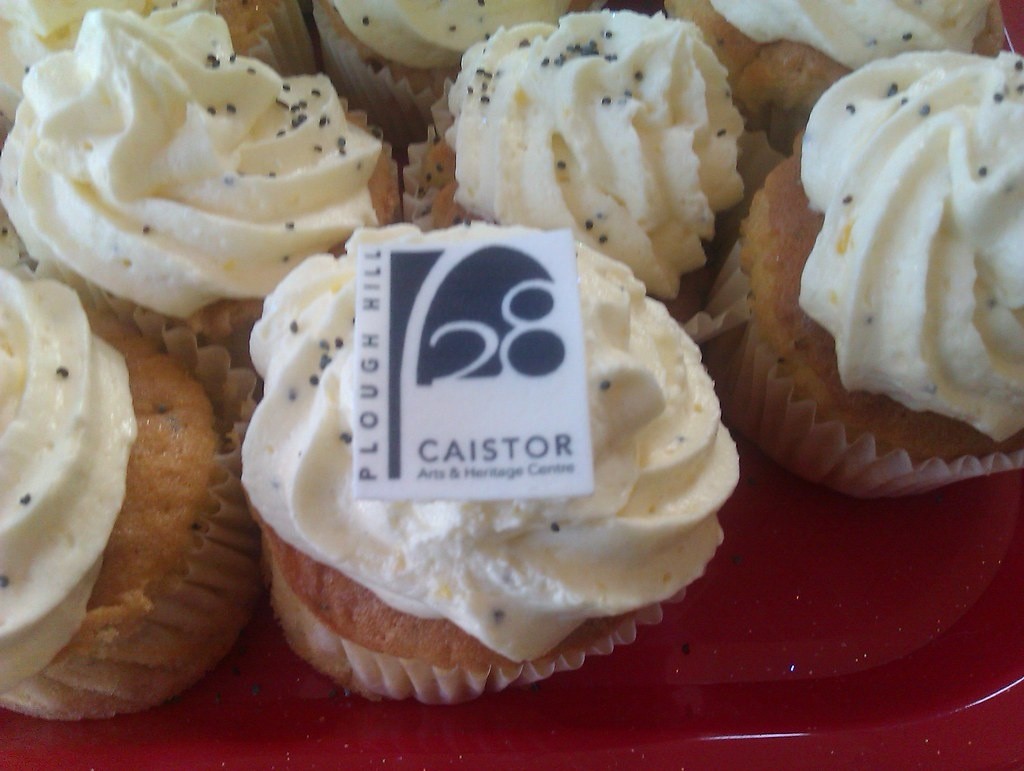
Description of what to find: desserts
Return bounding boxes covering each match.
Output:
[0,0,1024,727]
